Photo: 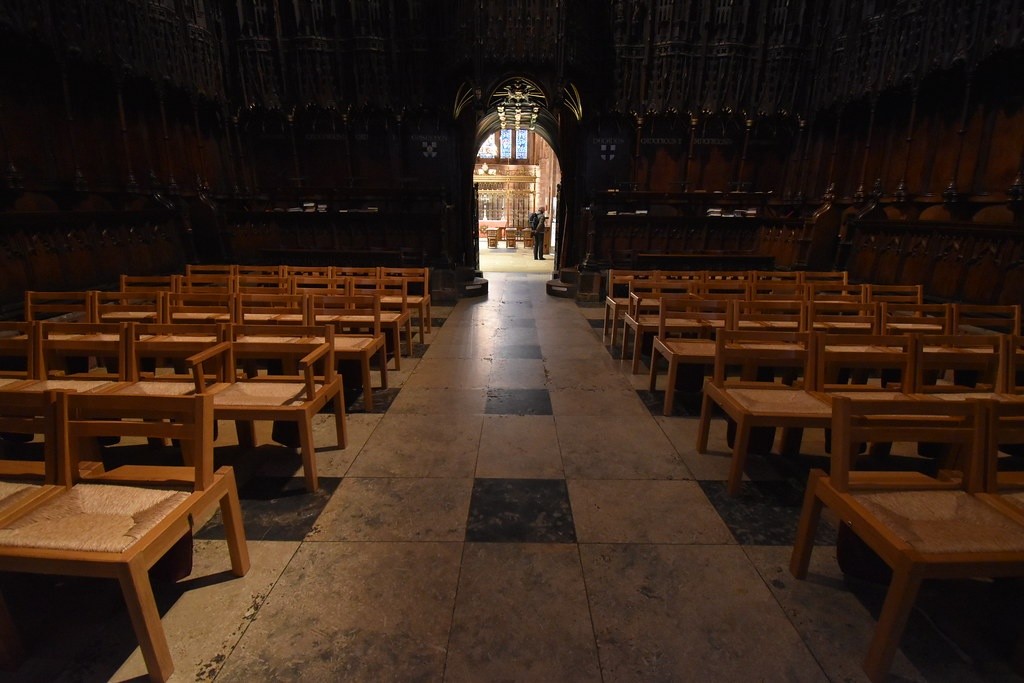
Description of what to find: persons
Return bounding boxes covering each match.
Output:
[529,206,546,260]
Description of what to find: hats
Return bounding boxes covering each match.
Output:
[538,207,545,211]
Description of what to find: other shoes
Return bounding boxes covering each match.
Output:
[540,258,546,260]
[534,258,539,260]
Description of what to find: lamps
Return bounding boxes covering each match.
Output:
[483,164,489,174]
[496,98,541,131]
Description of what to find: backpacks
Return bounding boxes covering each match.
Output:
[529,213,539,228]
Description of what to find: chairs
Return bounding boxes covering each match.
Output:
[605,269,1024,683]
[399,246,425,268]
[0,263,433,683]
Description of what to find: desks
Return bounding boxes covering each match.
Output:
[486,227,499,249]
[635,254,776,271]
[521,227,534,249]
[256,248,429,268]
[506,228,517,249]
[543,227,551,255]
